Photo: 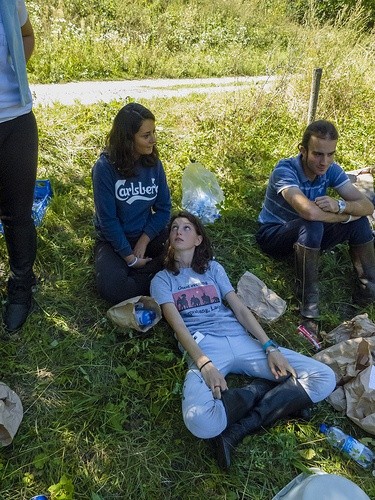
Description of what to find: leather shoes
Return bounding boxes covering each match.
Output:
[4,302,30,332]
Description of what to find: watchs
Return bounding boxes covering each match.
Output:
[263,340,279,351]
[336,199,346,214]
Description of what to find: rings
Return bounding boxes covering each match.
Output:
[214,385,221,388]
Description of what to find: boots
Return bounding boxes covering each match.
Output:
[293,241,321,318]
[347,236,375,307]
[221,378,310,429]
[215,375,314,470]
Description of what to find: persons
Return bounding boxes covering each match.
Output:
[256,120,375,318]
[0,0,39,330]
[149,210,336,471]
[91,102,172,304]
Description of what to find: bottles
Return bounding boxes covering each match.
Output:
[320,423,375,469]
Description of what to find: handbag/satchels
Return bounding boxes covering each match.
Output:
[0,179,53,234]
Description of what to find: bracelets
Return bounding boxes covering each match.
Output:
[128,257,138,267]
[341,215,352,224]
[265,347,280,355]
[199,360,212,372]
